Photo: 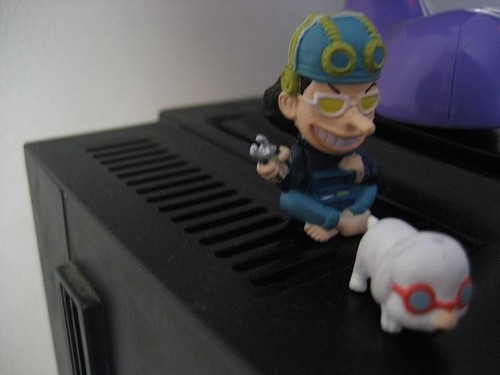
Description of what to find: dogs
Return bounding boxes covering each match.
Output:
[349,214,472,333]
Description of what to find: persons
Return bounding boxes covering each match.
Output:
[257,11,385,240]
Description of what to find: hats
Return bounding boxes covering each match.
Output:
[341,0,500,130]
